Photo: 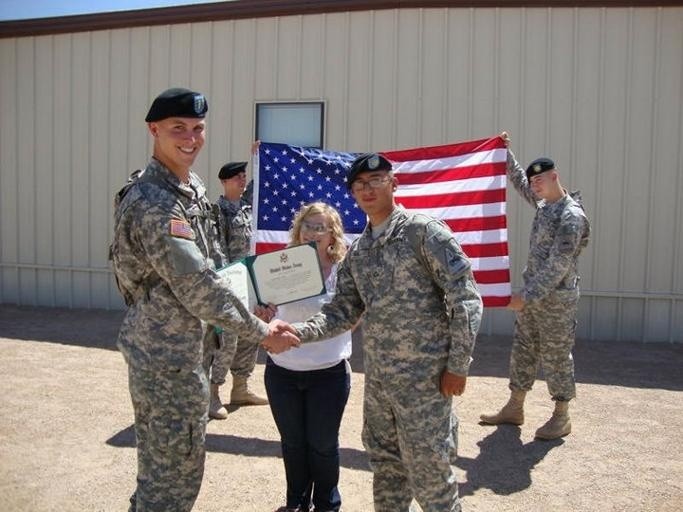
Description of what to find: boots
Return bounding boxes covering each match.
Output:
[209,383,229,420]
[536,400,571,439]
[480,389,527,425]
[229,375,270,406]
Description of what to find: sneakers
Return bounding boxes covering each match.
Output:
[277,504,313,512]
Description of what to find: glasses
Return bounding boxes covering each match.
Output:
[353,174,388,187]
[300,222,330,236]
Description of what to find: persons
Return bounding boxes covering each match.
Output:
[206,138,271,421]
[270,150,485,512]
[479,130,593,442]
[107,86,302,511]
[252,199,354,512]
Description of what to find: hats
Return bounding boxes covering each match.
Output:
[145,89,208,124]
[343,153,392,189]
[217,162,249,181]
[525,158,554,179]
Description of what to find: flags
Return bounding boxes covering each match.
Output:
[249,134,513,309]
